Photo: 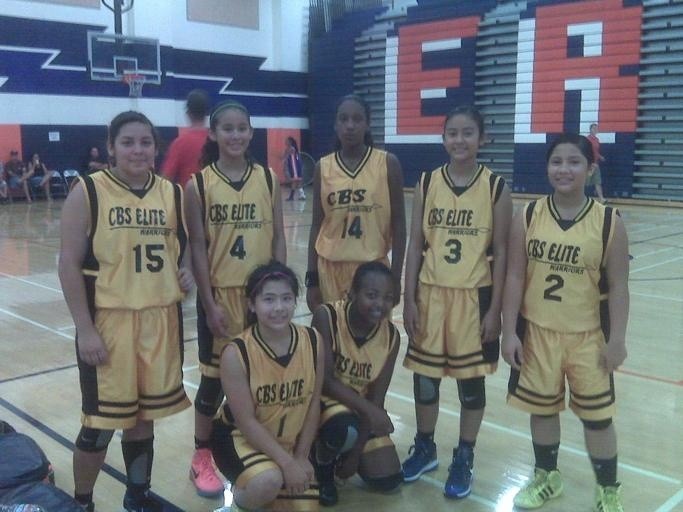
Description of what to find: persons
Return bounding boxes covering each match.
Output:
[308,260,404,506]
[1,150,54,204]
[286,136,306,201]
[156,89,212,184]
[210,258,326,512]
[185,99,286,496]
[501,132,630,512]
[585,124,608,205]
[404,107,513,499]
[305,96,405,314]
[58,111,193,511]
[82,146,105,169]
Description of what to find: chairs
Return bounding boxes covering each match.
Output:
[0,169,81,202]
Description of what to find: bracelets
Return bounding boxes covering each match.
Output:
[305,270,319,288]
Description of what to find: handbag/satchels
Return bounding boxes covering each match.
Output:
[3,481,78,512]
[1,417,55,489]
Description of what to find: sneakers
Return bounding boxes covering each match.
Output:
[187,445,228,501]
[589,479,627,511]
[120,483,167,512]
[400,436,440,483]
[315,461,340,507]
[73,498,97,512]
[511,465,566,510]
[442,443,476,500]
[598,198,608,204]
